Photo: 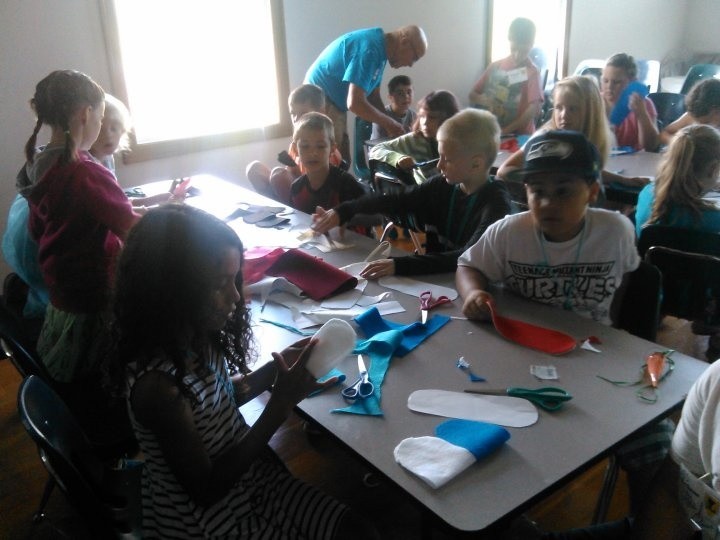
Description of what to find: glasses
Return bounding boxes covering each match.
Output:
[409,40,420,63]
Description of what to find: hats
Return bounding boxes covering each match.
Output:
[515,130,603,185]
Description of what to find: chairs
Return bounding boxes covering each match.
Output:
[0,273,105,540]
[529,48,720,152]
[365,160,720,347]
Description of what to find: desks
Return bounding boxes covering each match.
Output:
[120,172,712,535]
[490,135,720,210]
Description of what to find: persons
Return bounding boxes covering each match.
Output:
[3,17,720,540]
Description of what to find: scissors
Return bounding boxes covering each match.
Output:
[341,354,375,398]
[463,386,572,410]
[415,157,440,167]
[412,291,450,325]
[312,213,337,249]
[124,188,146,197]
